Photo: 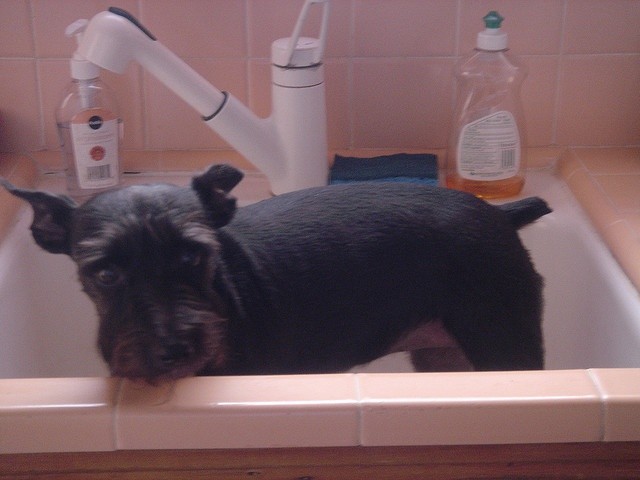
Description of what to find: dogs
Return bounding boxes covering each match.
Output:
[1,163,553,386]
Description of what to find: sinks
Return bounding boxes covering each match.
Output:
[0,183,639,454]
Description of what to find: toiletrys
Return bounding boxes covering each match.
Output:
[51,18,126,195]
[442,9,527,199]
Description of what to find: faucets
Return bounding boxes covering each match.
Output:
[81,2,337,197]
